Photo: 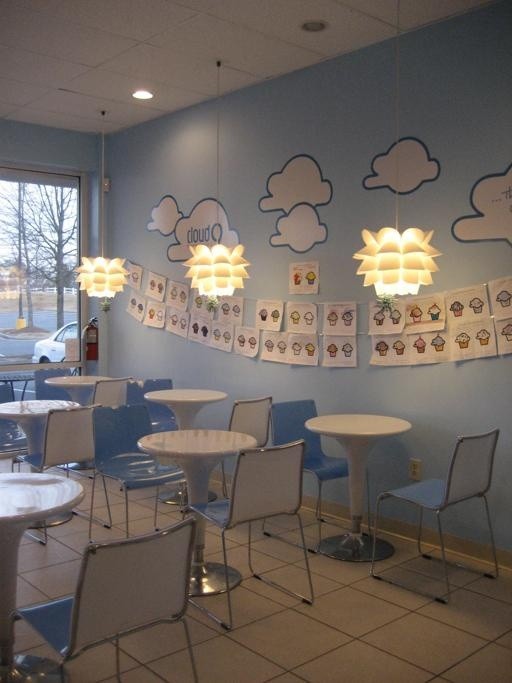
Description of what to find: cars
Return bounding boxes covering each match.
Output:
[28,318,80,375]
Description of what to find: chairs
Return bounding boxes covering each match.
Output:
[261,400,371,555]
[0,366,274,549]
[8,516,199,680]
[189,439,314,629]
[371,430,498,604]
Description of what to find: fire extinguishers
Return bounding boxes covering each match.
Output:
[81,317,98,361]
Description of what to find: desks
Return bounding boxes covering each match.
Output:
[137,429,257,598]
[2,472,86,681]
[304,414,412,562]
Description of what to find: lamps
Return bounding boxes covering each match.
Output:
[72,109,132,300]
[352,0,443,303]
[181,58,252,305]
